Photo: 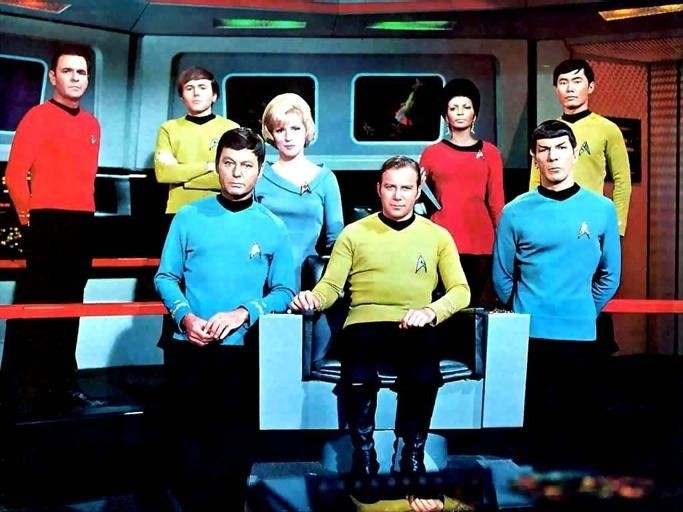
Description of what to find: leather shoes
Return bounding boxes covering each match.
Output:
[52,385,109,417]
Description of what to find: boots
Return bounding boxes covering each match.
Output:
[338,382,380,473]
[392,385,437,473]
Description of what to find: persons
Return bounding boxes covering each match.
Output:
[133,127,297,511]
[1,46,113,420]
[286,157,471,477]
[527,60,633,356]
[414,79,506,308]
[491,119,622,478]
[152,66,244,346]
[255,93,345,297]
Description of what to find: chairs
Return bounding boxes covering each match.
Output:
[257,255,531,474]
